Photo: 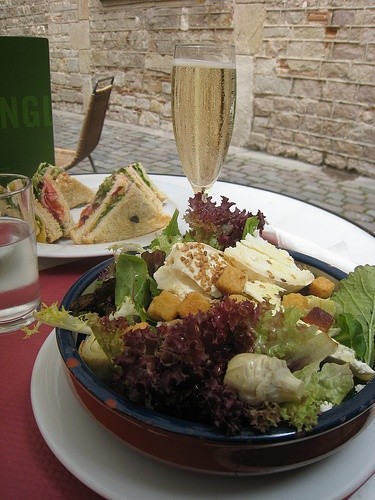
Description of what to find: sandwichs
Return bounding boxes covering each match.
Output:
[69,162,172,245]
[0,162,97,244]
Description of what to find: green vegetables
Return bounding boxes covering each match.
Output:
[18,188,375,438]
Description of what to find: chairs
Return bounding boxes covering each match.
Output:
[54,75,114,173]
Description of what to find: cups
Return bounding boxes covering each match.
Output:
[173,43,235,200]
[0,173,41,333]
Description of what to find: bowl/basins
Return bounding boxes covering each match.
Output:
[54,247,375,475]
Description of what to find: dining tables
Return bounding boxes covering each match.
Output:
[0,173,374,498]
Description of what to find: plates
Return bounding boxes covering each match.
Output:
[37,202,190,258]
[30,327,374,500]
